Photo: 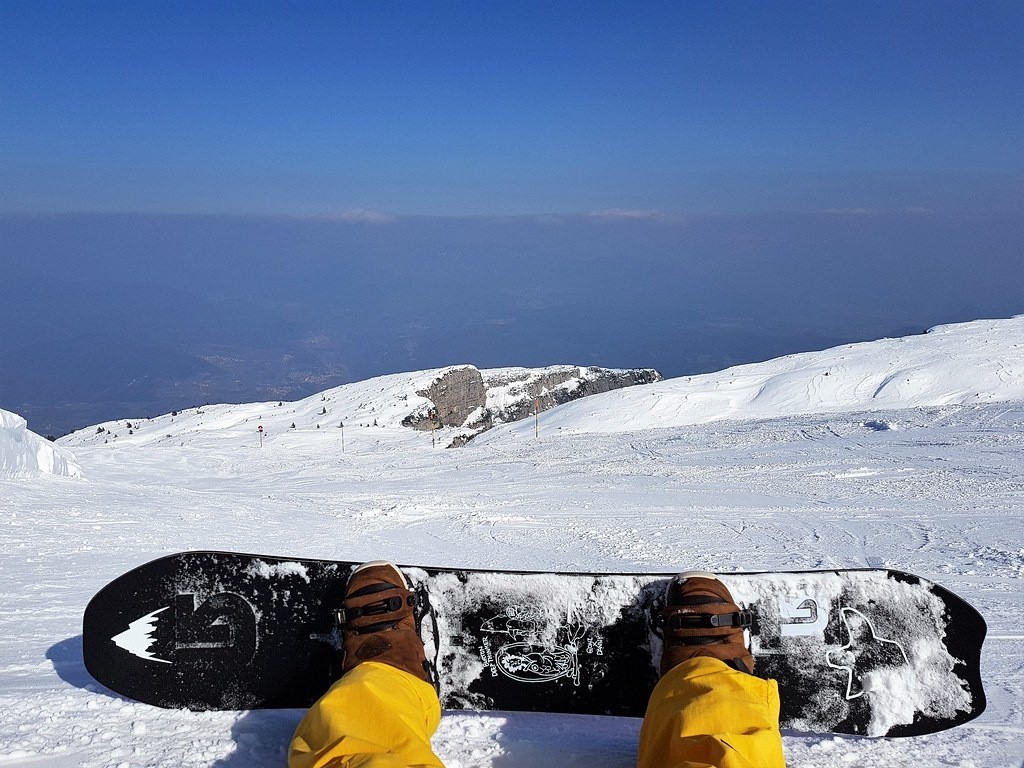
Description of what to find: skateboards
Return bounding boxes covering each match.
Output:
[82,548,988,743]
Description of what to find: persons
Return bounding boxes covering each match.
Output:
[288,559,786,768]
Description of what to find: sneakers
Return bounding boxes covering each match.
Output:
[339,561,429,684]
[661,572,754,680]
